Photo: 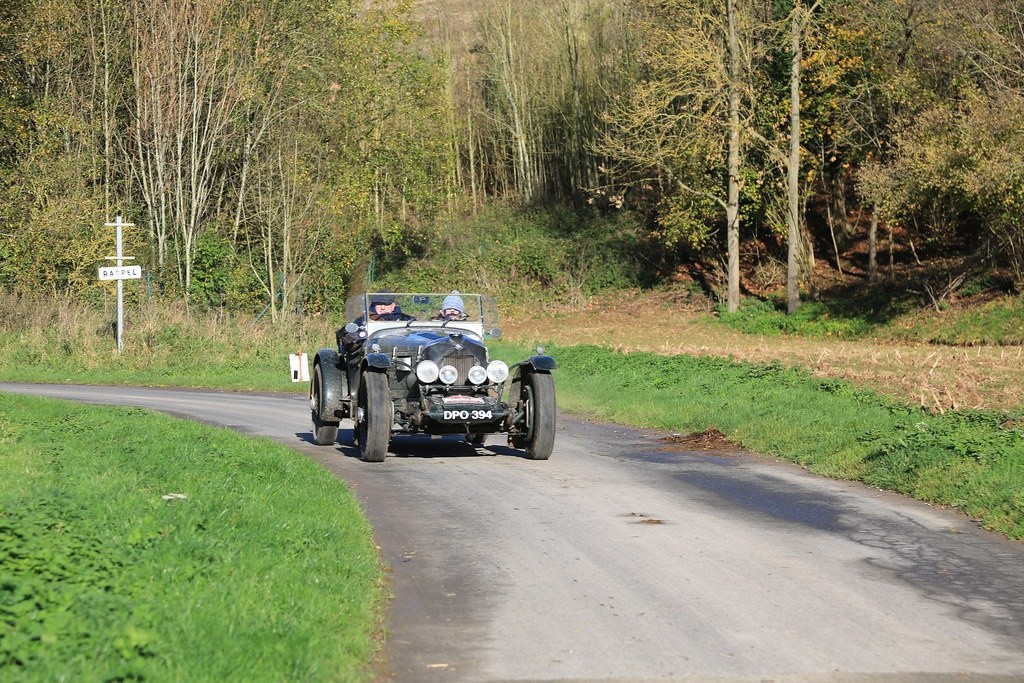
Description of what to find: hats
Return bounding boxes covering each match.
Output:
[372,289,395,305]
[441,290,464,315]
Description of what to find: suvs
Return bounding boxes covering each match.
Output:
[311,293,558,462]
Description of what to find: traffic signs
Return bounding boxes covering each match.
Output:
[98,265,142,280]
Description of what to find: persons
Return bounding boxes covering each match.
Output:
[366,290,409,323]
[436,290,469,321]
